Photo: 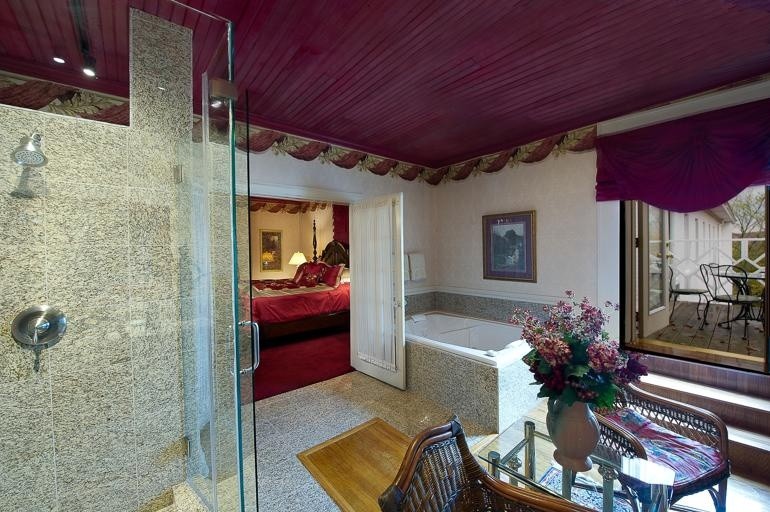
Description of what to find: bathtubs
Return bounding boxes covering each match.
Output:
[403,309,541,368]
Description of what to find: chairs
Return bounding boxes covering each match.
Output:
[668,262,707,326]
[699,263,763,340]
[596,383,730,512]
[379,415,602,512]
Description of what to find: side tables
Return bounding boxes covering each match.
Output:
[478,414,676,511]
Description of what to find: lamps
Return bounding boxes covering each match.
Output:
[289,252,307,267]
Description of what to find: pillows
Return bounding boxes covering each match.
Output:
[315,262,345,288]
[292,262,325,286]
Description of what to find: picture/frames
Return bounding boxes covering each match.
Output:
[260,230,282,272]
[482,210,537,283]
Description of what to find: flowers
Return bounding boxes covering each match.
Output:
[510,292,648,407]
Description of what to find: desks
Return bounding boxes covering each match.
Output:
[711,272,767,337]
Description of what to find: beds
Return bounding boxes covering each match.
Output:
[251,240,350,341]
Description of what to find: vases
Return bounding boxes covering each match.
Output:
[553,399,600,472]
